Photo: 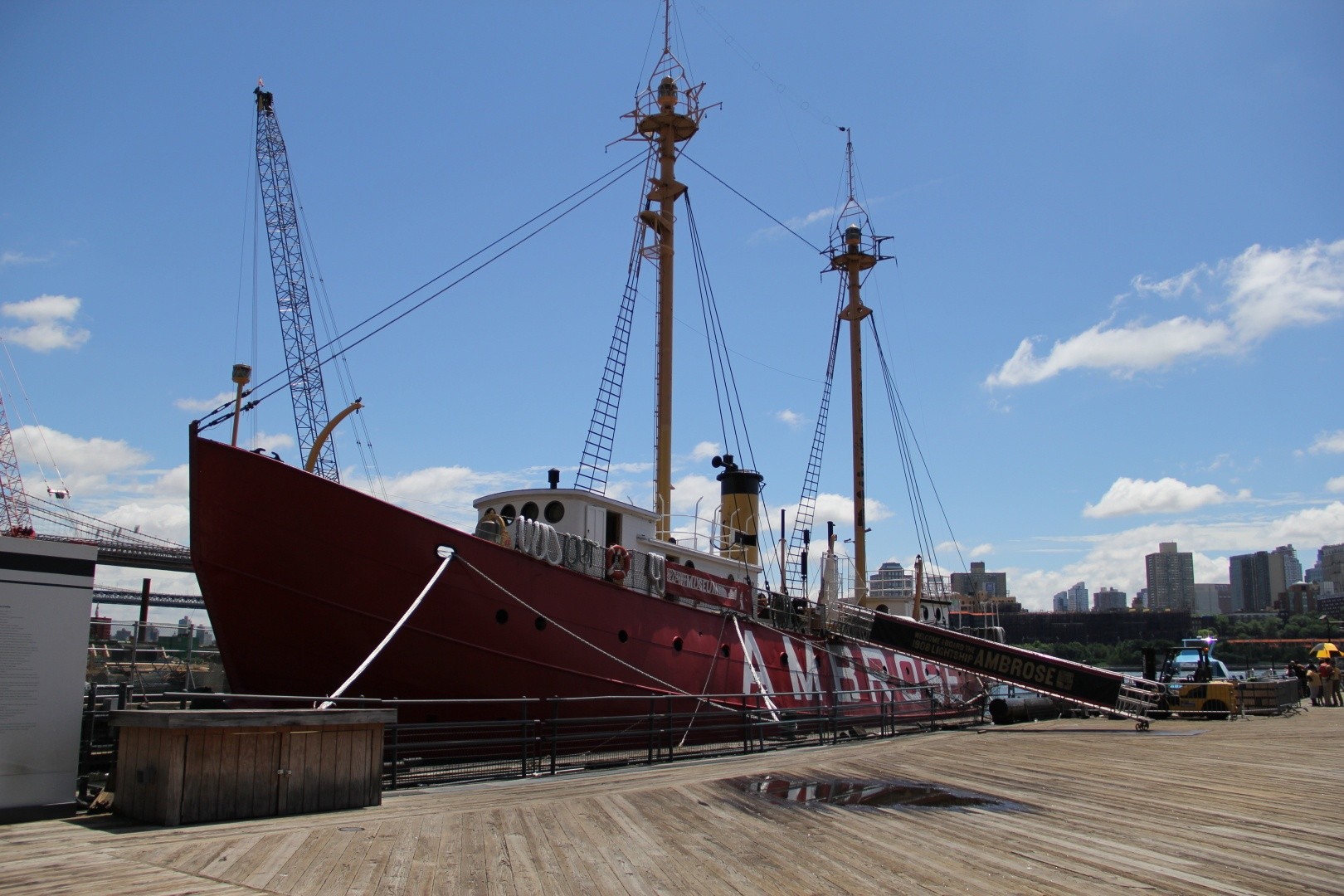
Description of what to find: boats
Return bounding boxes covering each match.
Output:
[188,0,989,758]
[1162,635,1230,682]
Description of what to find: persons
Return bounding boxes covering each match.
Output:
[1288,658,1344,707]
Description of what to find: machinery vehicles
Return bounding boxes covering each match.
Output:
[1138,645,1237,721]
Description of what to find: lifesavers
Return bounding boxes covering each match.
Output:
[606,544,631,580]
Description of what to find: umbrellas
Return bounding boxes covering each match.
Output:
[1309,643,1344,660]
